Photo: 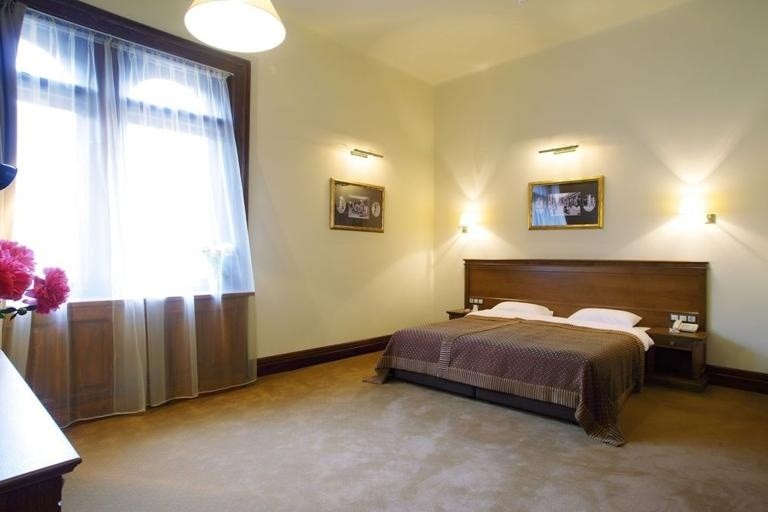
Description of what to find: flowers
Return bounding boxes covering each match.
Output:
[1,233,70,319]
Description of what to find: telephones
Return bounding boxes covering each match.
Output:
[672,320,699,333]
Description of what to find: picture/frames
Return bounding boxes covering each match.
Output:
[528,176,609,233]
[329,178,388,234]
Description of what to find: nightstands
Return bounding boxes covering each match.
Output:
[649,323,705,391]
[449,301,470,321]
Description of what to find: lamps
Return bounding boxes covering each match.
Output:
[180,0,290,58]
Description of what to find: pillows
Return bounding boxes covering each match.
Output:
[489,300,556,325]
[571,303,646,332]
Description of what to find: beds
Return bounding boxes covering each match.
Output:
[363,258,709,446]
